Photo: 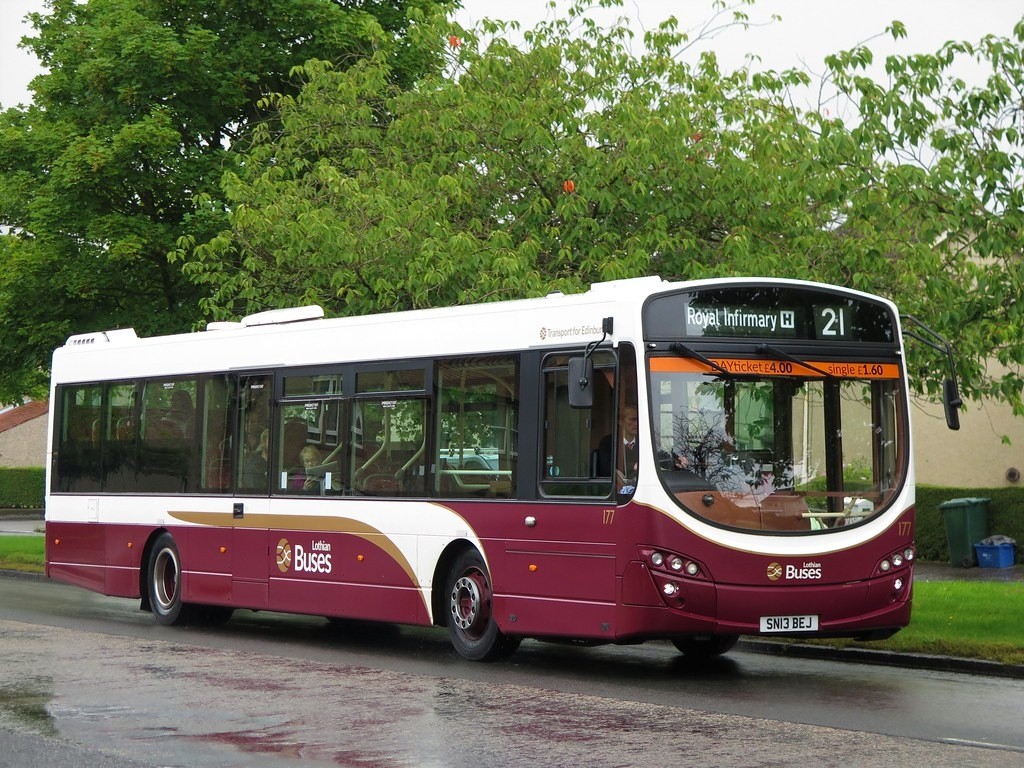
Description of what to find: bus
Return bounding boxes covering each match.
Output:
[42,273,963,666]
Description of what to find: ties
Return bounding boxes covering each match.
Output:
[626,442,634,449]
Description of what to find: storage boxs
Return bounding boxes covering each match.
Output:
[973,543,1014,568]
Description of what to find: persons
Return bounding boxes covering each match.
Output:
[671,372,737,469]
[159,390,197,444]
[288,445,341,495]
[283,415,308,495]
[595,405,689,498]
[237,375,271,494]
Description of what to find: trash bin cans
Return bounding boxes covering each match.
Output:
[938,496,994,568]
[973,534,1017,568]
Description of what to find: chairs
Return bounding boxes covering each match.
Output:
[208,458,230,489]
[116,416,134,440]
[146,415,186,440]
[363,475,399,490]
[589,449,601,496]
[91,417,118,443]
[322,472,342,495]
